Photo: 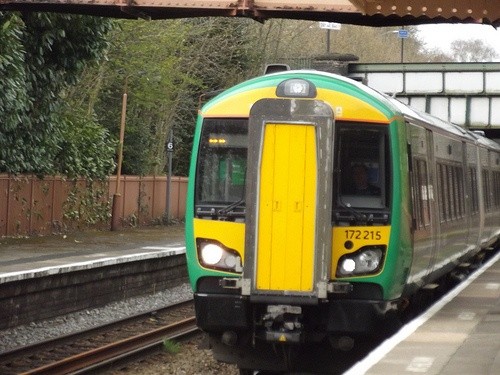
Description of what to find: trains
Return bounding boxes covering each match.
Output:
[185,66,499,374]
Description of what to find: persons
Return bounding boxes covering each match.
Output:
[342,165,381,196]
